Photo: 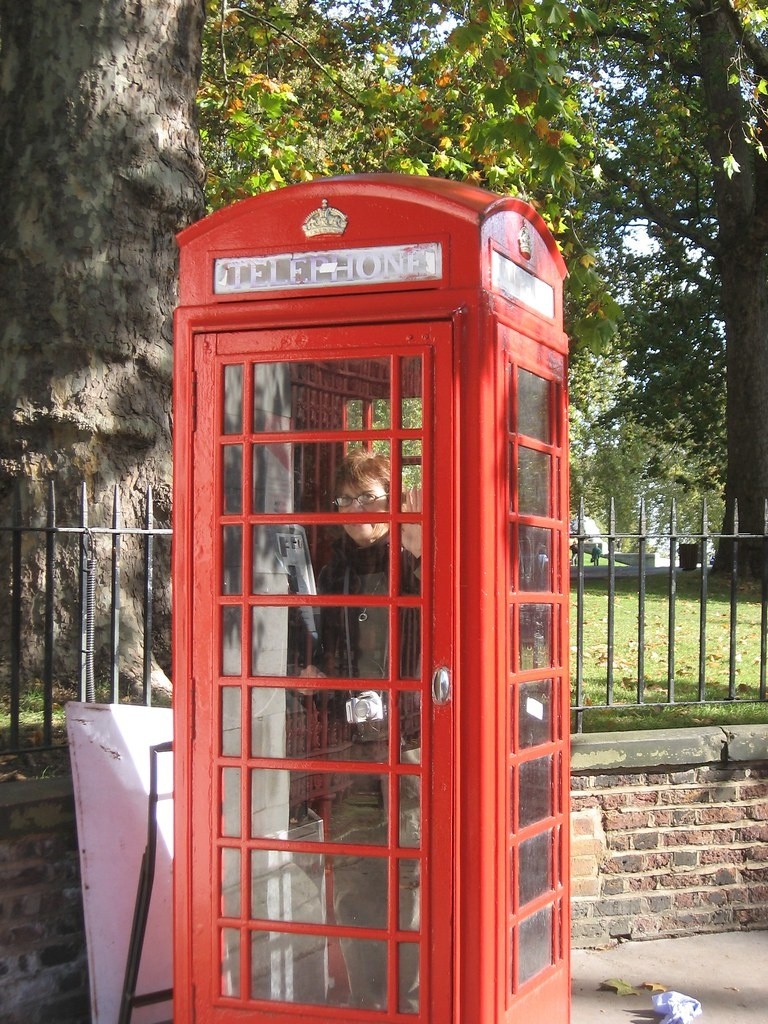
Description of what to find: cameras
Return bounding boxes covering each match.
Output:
[345,690,383,724]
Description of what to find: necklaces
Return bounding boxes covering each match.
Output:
[356,576,382,622]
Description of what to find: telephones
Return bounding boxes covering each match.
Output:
[254,524,325,663]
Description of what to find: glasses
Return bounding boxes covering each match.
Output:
[331,492,389,508]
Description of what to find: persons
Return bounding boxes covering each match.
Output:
[298,451,421,1012]
[592,544,602,565]
[570,540,578,566]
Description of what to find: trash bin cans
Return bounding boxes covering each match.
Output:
[678,544,700,571]
